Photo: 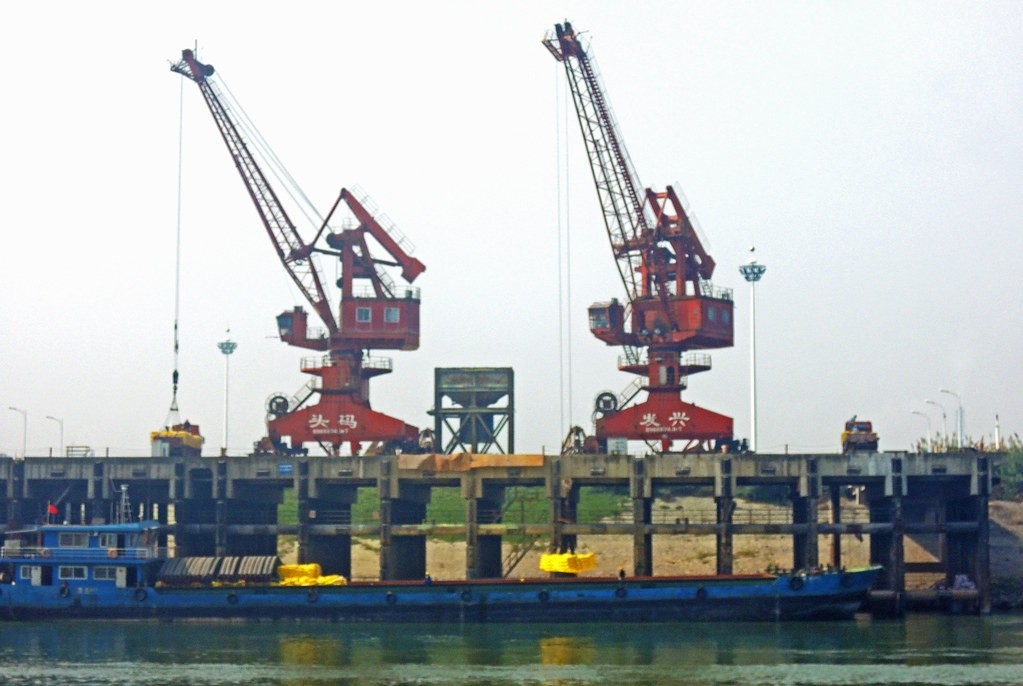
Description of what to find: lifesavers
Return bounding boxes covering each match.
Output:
[460,590,473,602]
[891,518,907,536]
[308,592,318,603]
[537,590,549,602]
[386,591,398,605]
[615,587,627,599]
[226,594,238,605]
[839,574,856,587]
[134,588,147,602]
[696,587,707,599]
[788,575,803,593]
[58,584,69,598]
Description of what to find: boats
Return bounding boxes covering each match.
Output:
[0,484,885,623]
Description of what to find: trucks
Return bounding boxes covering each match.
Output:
[841,422,879,453]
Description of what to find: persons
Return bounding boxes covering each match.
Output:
[618,566,626,580]
[795,566,823,581]
[848,413,860,433]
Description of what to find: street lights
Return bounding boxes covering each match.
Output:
[216,327,239,457]
[737,245,765,453]
[46,415,63,457]
[9,406,27,458]
[911,389,963,453]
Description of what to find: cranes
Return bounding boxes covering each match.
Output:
[542,16,747,454]
[150,38,425,456]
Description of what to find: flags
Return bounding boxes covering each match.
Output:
[48,505,58,514]
